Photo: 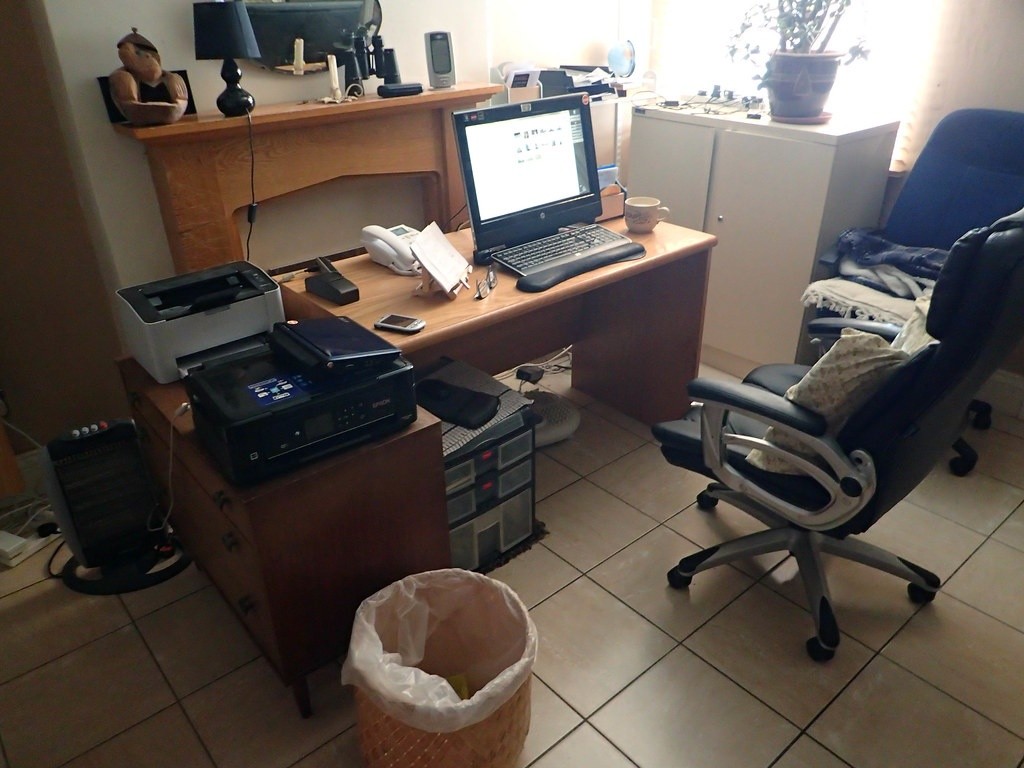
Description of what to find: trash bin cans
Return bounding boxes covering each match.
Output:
[340,569,538,768]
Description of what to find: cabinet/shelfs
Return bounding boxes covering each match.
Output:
[113,353,454,725]
[623,111,900,382]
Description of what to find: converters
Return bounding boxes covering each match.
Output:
[516,365,545,383]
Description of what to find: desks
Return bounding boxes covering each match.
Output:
[274,216,718,426]
[113,83,504,277]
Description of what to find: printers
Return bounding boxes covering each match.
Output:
[184,316,418,489]
[113,260,286,384]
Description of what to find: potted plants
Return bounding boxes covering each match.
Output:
[730,0,870,125]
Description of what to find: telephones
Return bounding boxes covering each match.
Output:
[361,224,423,275]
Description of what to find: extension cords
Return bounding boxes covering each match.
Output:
[0,530,61,567]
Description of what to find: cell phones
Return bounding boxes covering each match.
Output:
[374,314,426,333]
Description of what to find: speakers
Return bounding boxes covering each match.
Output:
[45,420,168,569]
[424,31,457,90]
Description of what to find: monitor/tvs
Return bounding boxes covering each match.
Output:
[447,90,603,252]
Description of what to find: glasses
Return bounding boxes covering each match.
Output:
[474,265,497,300]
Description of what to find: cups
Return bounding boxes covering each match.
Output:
[625,197,670,233]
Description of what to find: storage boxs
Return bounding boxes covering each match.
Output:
[595,179,628,223]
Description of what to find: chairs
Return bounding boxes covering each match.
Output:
[652,211,1024,664]
[817,109,1024,475]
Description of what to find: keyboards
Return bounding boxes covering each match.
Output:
[491,224,631,277]
